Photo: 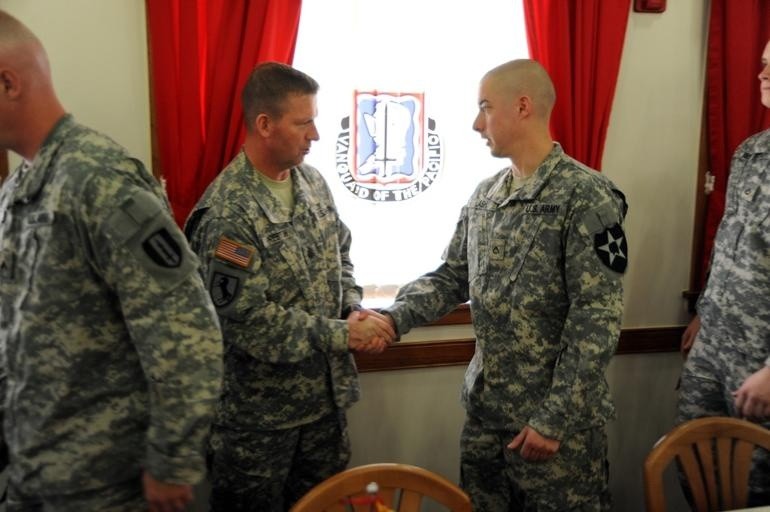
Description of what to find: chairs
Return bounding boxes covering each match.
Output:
[643,415,769,511]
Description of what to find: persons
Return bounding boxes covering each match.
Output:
[184,60,399,510]
[361,59,632,512]
[0,9,224,509]
[668,40,770,508]
